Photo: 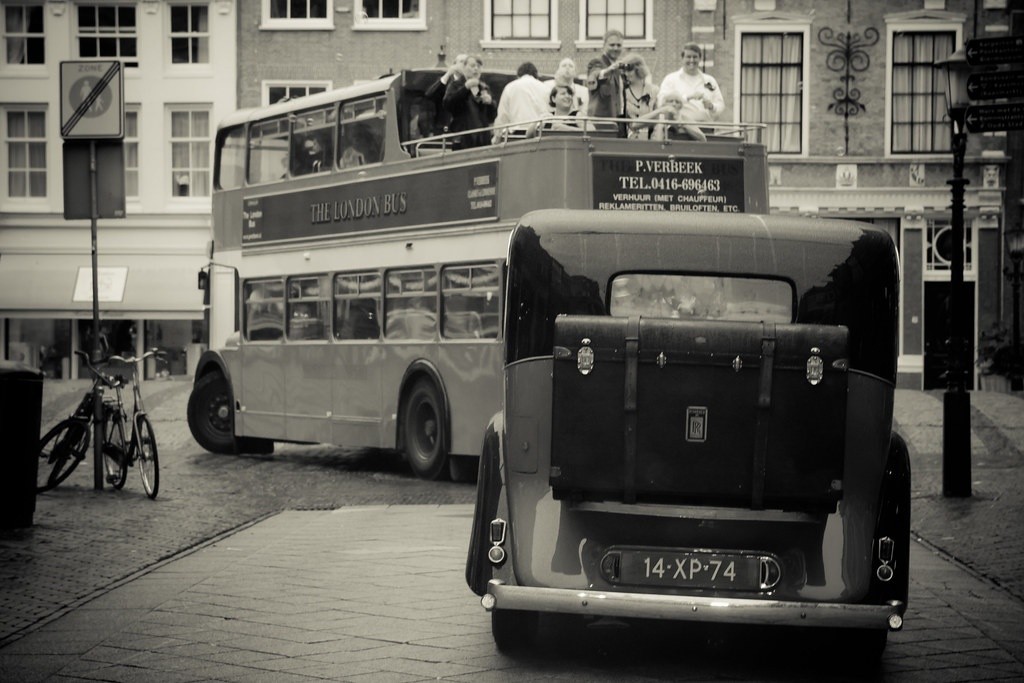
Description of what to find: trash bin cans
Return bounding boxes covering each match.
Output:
[0,368,46,532]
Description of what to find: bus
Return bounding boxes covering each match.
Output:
[188,68,769,484]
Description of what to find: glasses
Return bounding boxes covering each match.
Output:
[303,138,317,152]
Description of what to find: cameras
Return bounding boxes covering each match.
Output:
[618,63,632,70]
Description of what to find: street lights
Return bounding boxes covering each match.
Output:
[922,48,975,501]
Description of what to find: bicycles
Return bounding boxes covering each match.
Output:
[98,347,167,499]
[24,350,119,493]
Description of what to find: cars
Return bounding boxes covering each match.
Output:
[466,206,906,665]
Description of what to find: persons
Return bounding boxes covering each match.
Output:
[295,134,332,175]
[410,30,726,151]
[39,344,62,379]
